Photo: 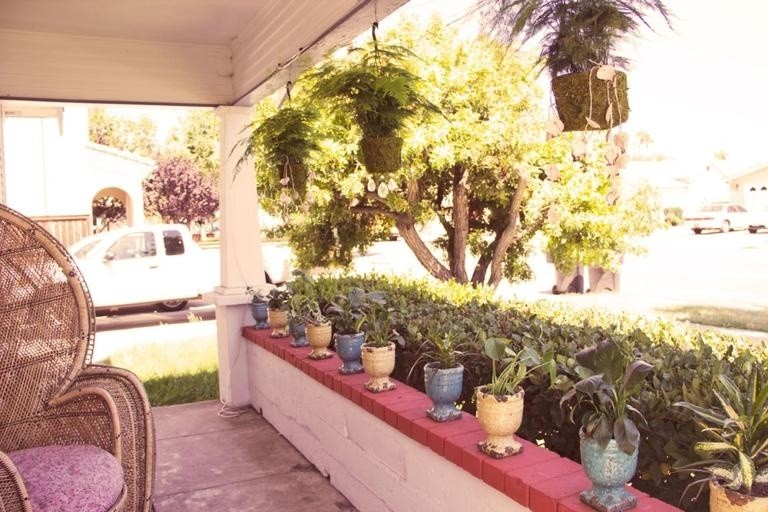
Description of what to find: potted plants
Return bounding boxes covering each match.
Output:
[409,323,473,421]
[250,287,271,331]
[359,303,398,391]
[553,326,663,512]
[672,363,768,511]
[333,289,377,373]
[303,302,340,359]
[495,1,667,131]
[313,43,435,174]
[231,106,317,189]
[475,334,558,458]
[265,289,292,338]
[284,295,309,347]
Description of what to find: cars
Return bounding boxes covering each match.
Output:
[4,223,215,325]
[683,202,750,234]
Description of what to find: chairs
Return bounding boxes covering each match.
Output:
[0,204,155,512]
